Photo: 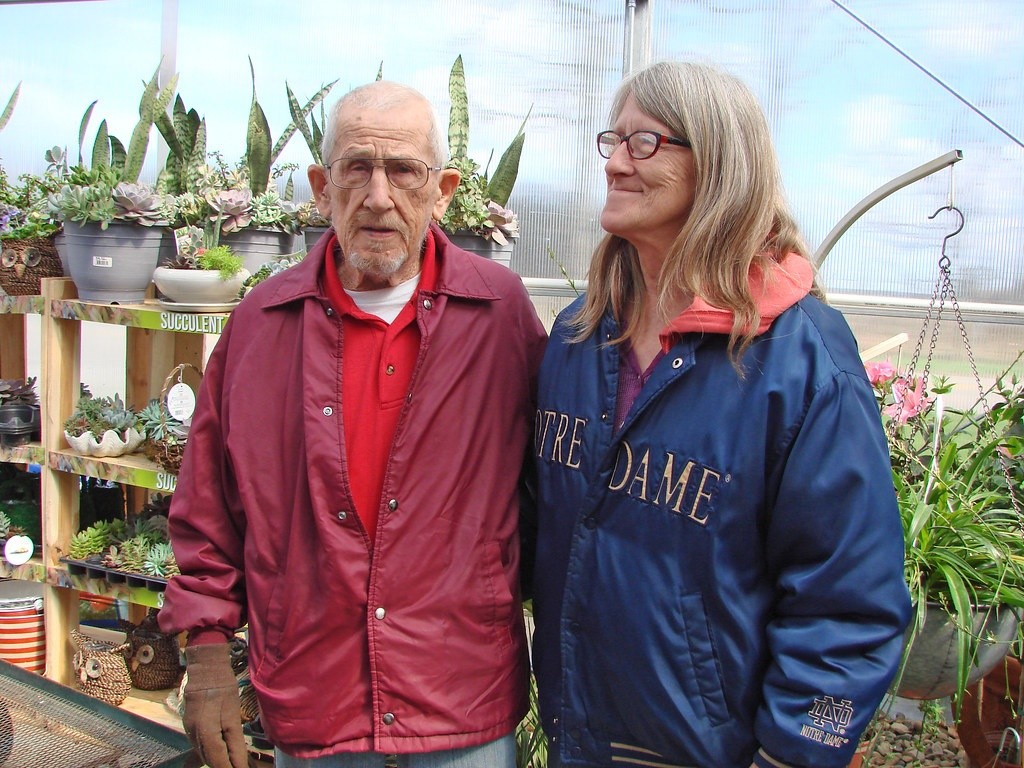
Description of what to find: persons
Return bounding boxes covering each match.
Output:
[532,61,912,768]
[156,81,549,768]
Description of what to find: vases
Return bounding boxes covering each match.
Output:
[0,237,64,295]
[846,725,877,768]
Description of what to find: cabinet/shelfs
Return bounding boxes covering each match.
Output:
[0,274,276,768]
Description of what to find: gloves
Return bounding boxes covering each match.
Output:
[180,643,252,768]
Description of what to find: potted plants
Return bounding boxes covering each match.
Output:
[863,353,1024,703]
[0,376,40,423]
[0,511,29,556]
[59,383,189,592]
[46,53,535,312]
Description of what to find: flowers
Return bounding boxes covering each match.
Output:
[863,360,931,466]
[0,158,58,238]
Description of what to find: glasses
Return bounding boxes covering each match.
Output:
[324,157,442,191]
[596,128,694,161]
